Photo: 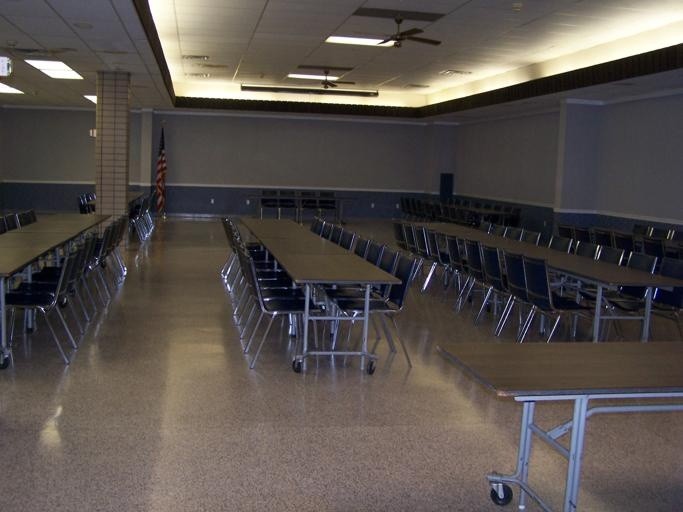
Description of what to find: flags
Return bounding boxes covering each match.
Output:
[153,126,169,215]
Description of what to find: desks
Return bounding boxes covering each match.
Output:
[434,340,683,511]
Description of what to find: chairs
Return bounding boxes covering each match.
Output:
[76,189,158,243]
[0,208,129,370]
[221,189,418,375]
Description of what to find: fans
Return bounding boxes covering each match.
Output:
[321,70,355,89]
[377,13,442,48]
